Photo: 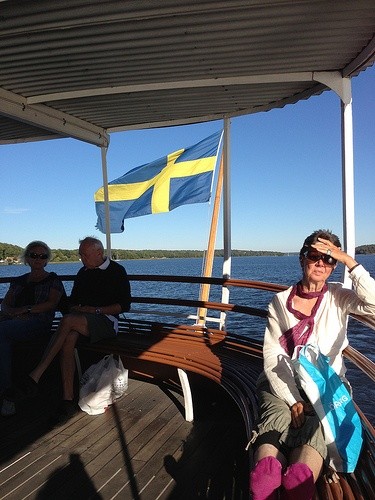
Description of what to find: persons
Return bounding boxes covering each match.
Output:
[0,241,63,417]
[245,229,375,500]
[3,237,131,422]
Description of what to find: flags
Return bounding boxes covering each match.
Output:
[93,129,224,233]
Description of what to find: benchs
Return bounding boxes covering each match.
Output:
[0,275,375,500]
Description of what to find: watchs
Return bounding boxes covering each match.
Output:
[27,304,32,313]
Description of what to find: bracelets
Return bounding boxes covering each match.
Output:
[96,306,101,315]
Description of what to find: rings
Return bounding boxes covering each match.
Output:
[327,249,331,254]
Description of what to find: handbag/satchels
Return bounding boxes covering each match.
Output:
[78,355,113,416]
[290,344,364,473]
[110,353,129,400]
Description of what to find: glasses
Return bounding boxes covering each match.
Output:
[304,250,338,265]
[26,253,49,259]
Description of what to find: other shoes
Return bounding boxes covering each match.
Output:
[48,399,77,429]
[0,399,17,416]
[9,374,39,398]
